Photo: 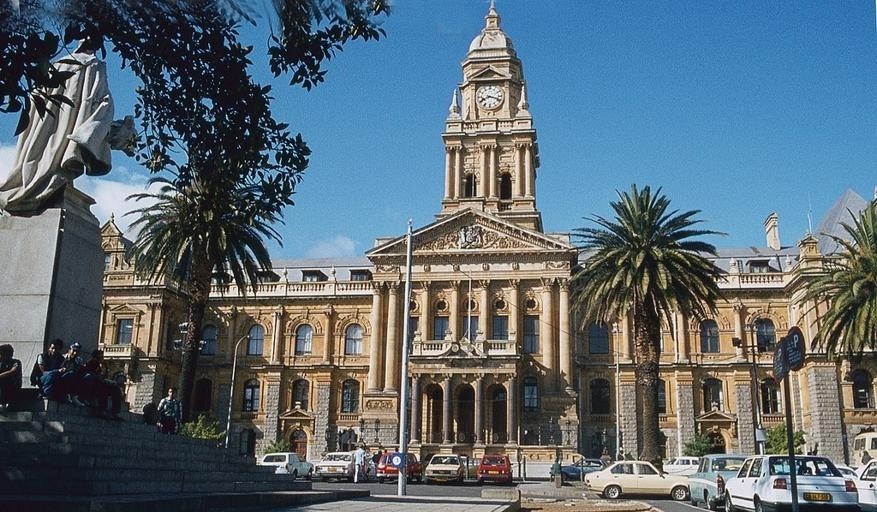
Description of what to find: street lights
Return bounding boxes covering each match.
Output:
[172,321,206,411]
[732,322,772,454]
[221,328,251,451]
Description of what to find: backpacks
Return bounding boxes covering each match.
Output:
[30,354,44,386]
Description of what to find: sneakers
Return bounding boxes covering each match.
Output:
[110,415,125,421]
[70,395,86,407]
[64,393,73,403]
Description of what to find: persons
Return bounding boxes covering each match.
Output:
[0,33,115,212]
[550,460,562,482]
[36,339,125,420]
[156,387,183,434]
[861,451,870,465]
[354,445,367,483]
[0,345,22,408]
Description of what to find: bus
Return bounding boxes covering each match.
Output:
[845,432,877,469]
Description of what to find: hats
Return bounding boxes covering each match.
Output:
[69,341,82,353]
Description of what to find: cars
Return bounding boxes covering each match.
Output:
[547,458,609,481]
[375,452,423,484]
[423,454,465,485]
[258,451,314,480]
[663,453,877,512]
[315,449,374,480]
[582,461,691,501]
[474,453,516,486]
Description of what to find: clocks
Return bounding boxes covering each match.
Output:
[475,82,505,112]
